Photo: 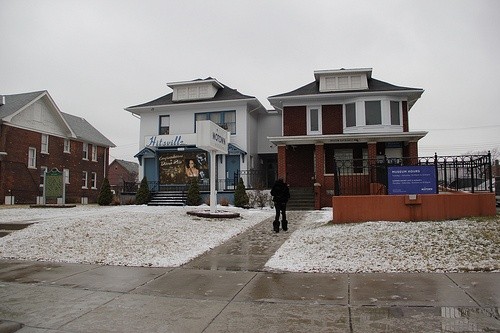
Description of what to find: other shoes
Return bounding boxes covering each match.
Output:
[282,220,288,231]
[273,221,280,233]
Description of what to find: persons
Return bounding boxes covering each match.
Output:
[270,177,290,233]
[184,158,199,178]
